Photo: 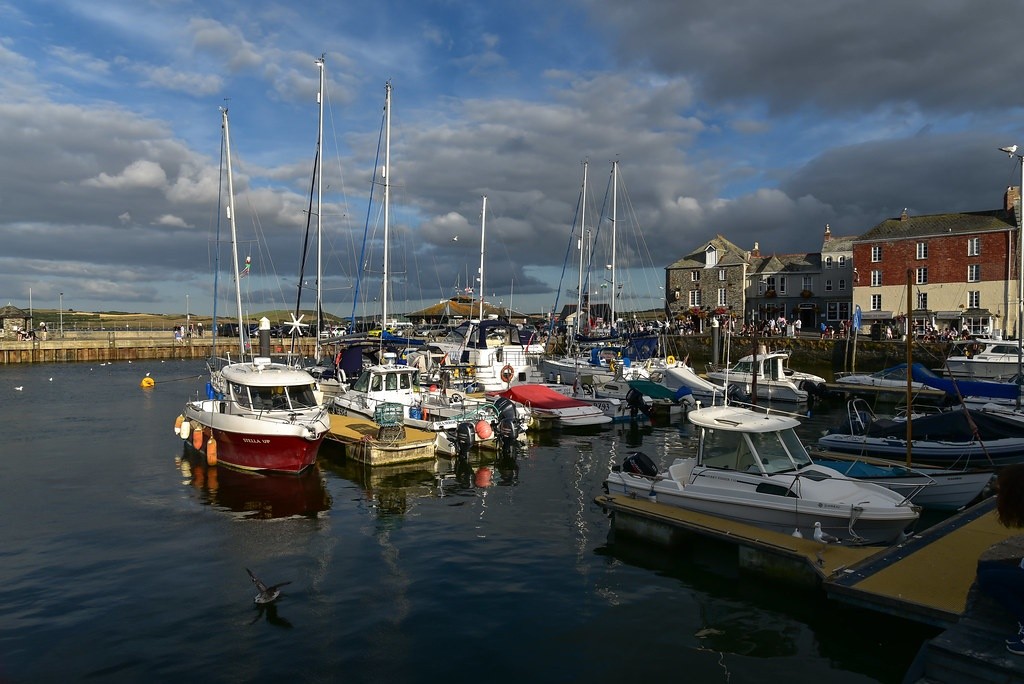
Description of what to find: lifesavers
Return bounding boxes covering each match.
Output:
[667,355,675,365]
[337,368,346,383]
[610,361,616,373]
[501,365,514,382]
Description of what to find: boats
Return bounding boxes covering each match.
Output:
[176,441,334,522]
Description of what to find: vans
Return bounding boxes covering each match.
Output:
[386,323,413,337]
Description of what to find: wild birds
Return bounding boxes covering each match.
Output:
[48,378,53,381]
[181,358,186,362]
[100,357,133,367]
[90,367,92,371]
[161,360,166,363]
[14,385,24,391]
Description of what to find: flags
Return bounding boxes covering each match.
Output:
[335,353,342,365]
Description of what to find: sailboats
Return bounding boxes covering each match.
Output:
[17,288,36,336]
[161,97,332,476]
[286,54,1022,627]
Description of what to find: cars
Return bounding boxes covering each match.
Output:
[414,324,446,337]
[368,325,395,338]
[319,327,347,340]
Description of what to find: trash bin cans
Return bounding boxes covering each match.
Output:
[869,322,886,341]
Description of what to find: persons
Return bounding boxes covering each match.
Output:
[595,320,695,337]
[885,325,959,344]
[963,343,983,355]
[610,358,616,372]
[217,324,223,336]
[982,326,990,339]
[820,319,848,339]
[740,315,802,337]
[422,318,426,324]
[961,321,969,340]
[17,324,47,342]
[173,323,203,339]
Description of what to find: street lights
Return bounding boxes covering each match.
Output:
[59,291,65,339]
[186,294,190,332]
